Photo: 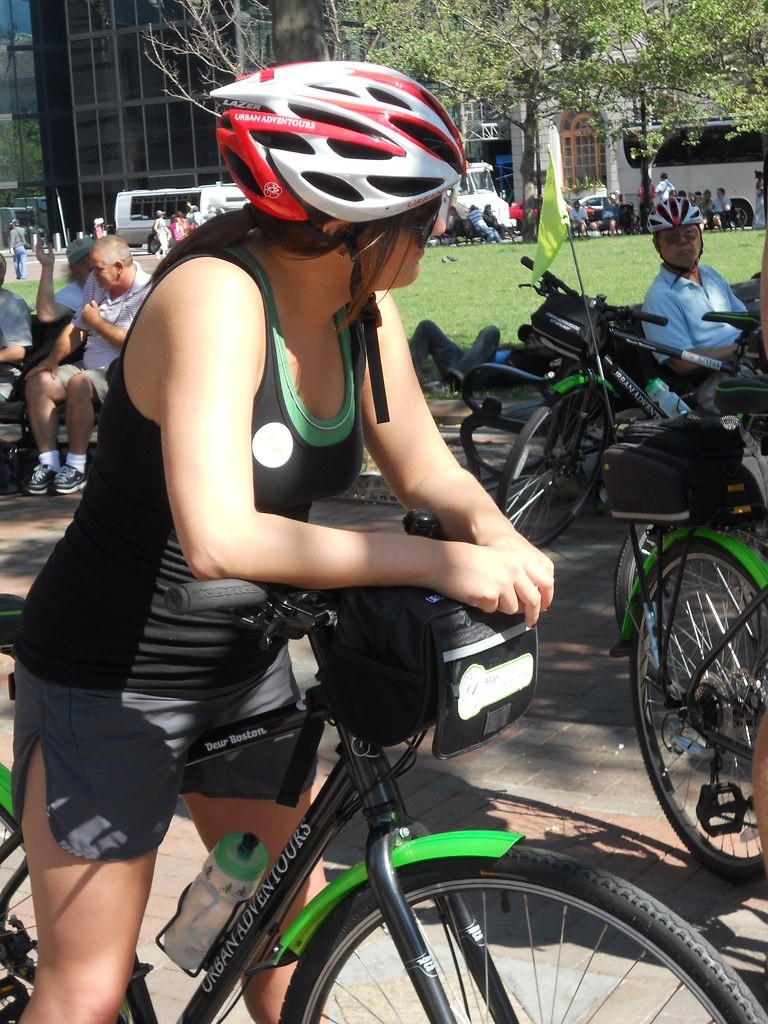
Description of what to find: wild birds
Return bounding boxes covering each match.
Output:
[441,255,459,263]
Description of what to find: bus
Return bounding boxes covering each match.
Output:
[616,114,768,227]
[115,184,250,257]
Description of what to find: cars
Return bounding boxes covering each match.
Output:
[506,193,577,222]
[575,195,608,213]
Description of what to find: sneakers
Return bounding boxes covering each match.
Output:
[24,464,57,494]
[51,463,87,494]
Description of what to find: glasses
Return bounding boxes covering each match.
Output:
[656,227,701,245]
[375,197,442,249]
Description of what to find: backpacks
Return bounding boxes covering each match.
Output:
[456,218,475,239]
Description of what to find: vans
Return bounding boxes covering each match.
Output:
[450,162,516,236]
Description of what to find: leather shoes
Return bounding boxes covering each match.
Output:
[424,380,457,398]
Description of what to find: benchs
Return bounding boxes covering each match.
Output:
[454,219,484,245]
[0,315,100,438]
[631,279,761,385]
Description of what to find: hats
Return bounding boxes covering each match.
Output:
[66,237,95,263]
[156,210,165,217]
[9,219,19,226]
[93,218,104,228]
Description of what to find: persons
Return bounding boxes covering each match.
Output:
[752,170,765,229]
[93,218,104,241]
[8,219,30,280]
[440,172,732,243]
[153,202,226,259]
[0,234,151,494]
[642,196,762,414]
[13,60,555,1023]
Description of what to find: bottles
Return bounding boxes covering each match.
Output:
[165,830,270,968]
[645,376,690,419]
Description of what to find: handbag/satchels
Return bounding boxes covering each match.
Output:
[601,414,753,515]
[24,243,30,249]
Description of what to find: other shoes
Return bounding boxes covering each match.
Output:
[155,251,159,260]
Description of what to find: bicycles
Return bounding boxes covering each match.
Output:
[463,250,767,861]
[0,515,768,1024]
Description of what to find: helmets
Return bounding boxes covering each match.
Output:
[647,196,703,231]
[210,61,466,222]
